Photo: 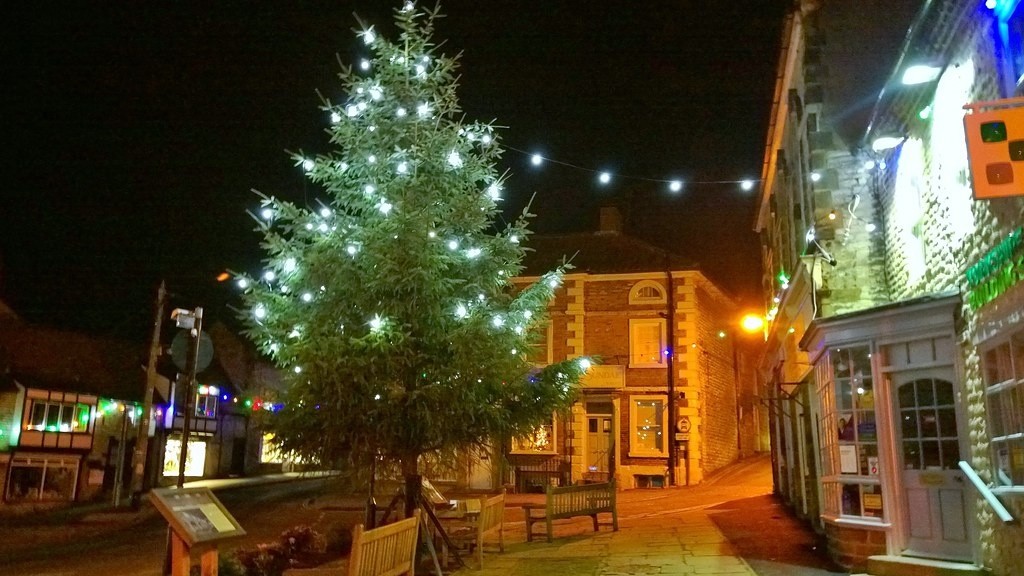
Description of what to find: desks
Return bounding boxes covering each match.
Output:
[434,510,463,552]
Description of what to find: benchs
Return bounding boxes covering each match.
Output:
[522,478,619,543]
[442,488,507,570]
[282,508,423,576]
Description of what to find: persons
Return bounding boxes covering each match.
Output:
[838,418,845,437]
